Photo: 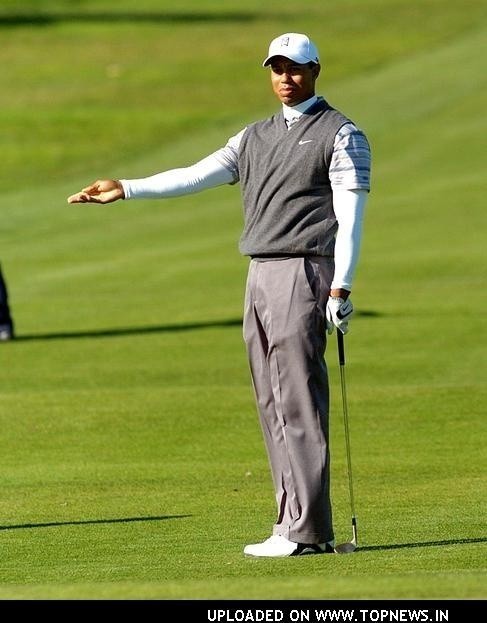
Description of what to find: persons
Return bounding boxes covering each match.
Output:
[0,264,13,341]
[67,33,372,557]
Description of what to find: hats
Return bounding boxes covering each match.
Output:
[262,32,319,67]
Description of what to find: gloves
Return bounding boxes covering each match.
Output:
[326,295,353,335]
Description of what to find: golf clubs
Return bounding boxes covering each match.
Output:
[331,323,360,554]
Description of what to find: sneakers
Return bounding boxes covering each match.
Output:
[244,533,334,556]
